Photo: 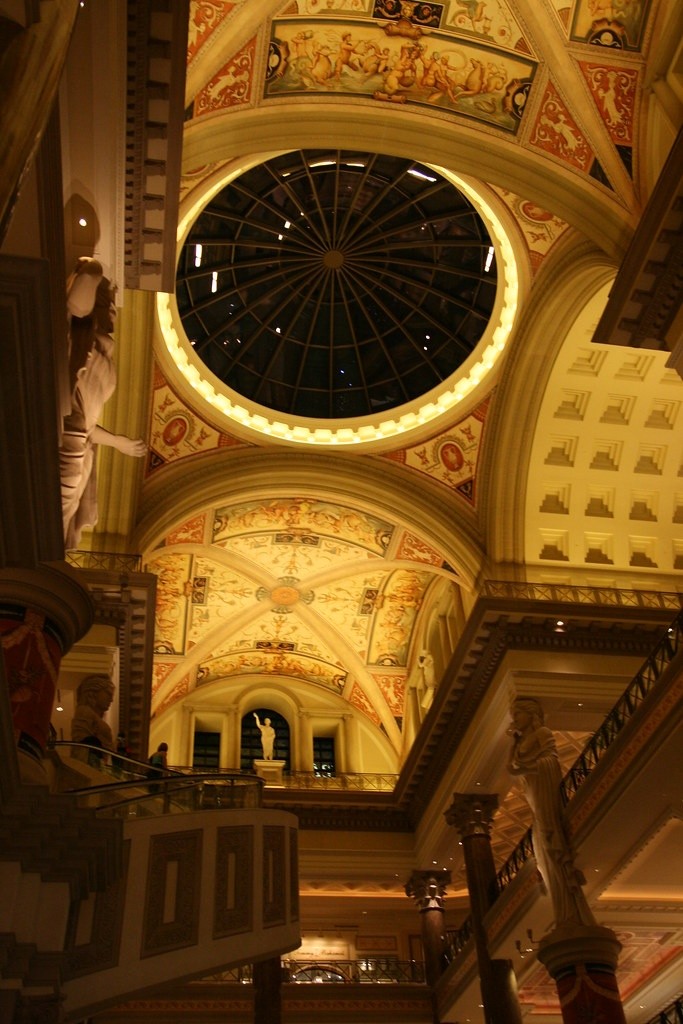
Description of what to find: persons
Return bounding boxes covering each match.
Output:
[439,935,453,973]
[111,732,127,779]
[416,650,435,687]
[70,673,115,772]
[148,742,168,793]
[254,713,276,760]
[60,256,147,548]
[506,699,596,926]
